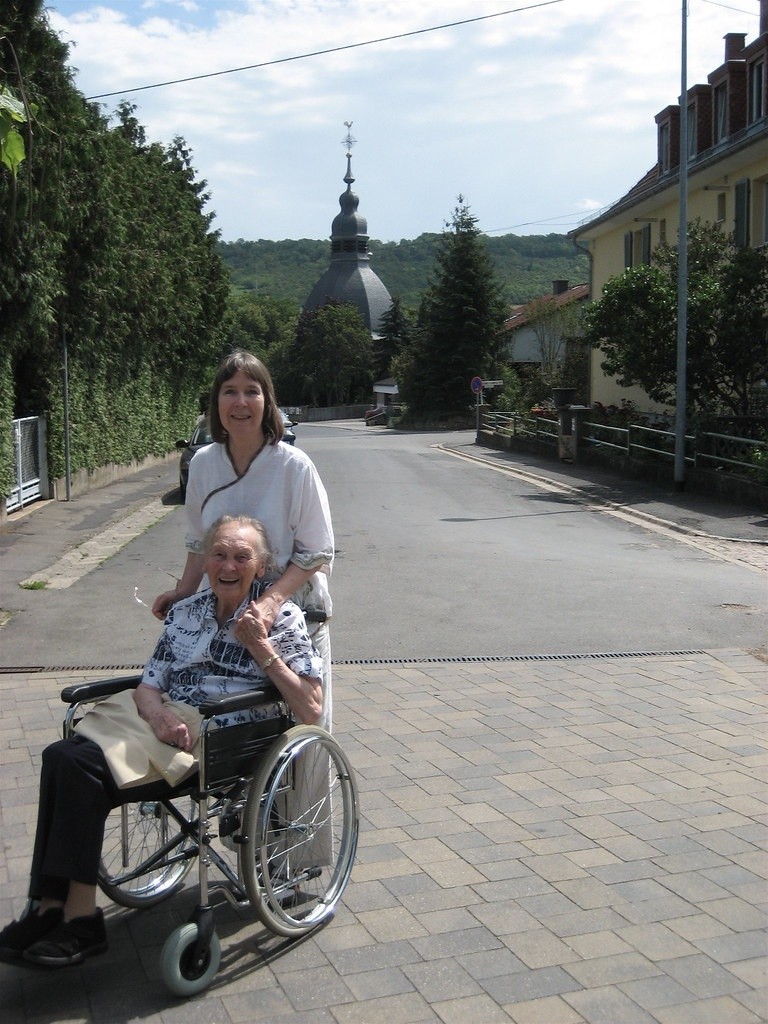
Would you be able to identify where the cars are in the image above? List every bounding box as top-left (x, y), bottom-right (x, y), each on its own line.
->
top-left (175, 409), bottom-right (298, 501)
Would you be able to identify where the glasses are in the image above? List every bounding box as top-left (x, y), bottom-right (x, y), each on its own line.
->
top-left (133, 561), bottom-right (184, 616)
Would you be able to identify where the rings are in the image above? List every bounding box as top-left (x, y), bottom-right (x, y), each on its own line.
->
top-left (170, 742), bottom-right (175, 746)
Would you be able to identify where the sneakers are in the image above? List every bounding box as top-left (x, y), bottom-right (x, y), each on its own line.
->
top-left (1, 907), bottom-right (64, 962)
top-left (24, 906), bottom-right (110, 966)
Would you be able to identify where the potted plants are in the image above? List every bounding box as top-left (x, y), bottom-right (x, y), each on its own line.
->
top-left (549, 365), bottom-right (577, 405)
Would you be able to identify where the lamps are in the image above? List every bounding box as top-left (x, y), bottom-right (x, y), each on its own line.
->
top-left (703, 185), bottom-right (731, 191)
top-left (634, 217), bottom-right (658, 222)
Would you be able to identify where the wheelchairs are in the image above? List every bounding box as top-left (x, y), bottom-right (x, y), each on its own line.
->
top-left (62, 611), bottom-right (361, 999)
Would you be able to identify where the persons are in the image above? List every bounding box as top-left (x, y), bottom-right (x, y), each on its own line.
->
top-left (152, 349), bottom-right (335, 908)
top-left (0, 512), bottom-right (323, 968)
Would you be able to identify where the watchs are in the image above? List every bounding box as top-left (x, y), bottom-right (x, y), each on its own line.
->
top-left (261, 654), bottom-right (280, 671)
